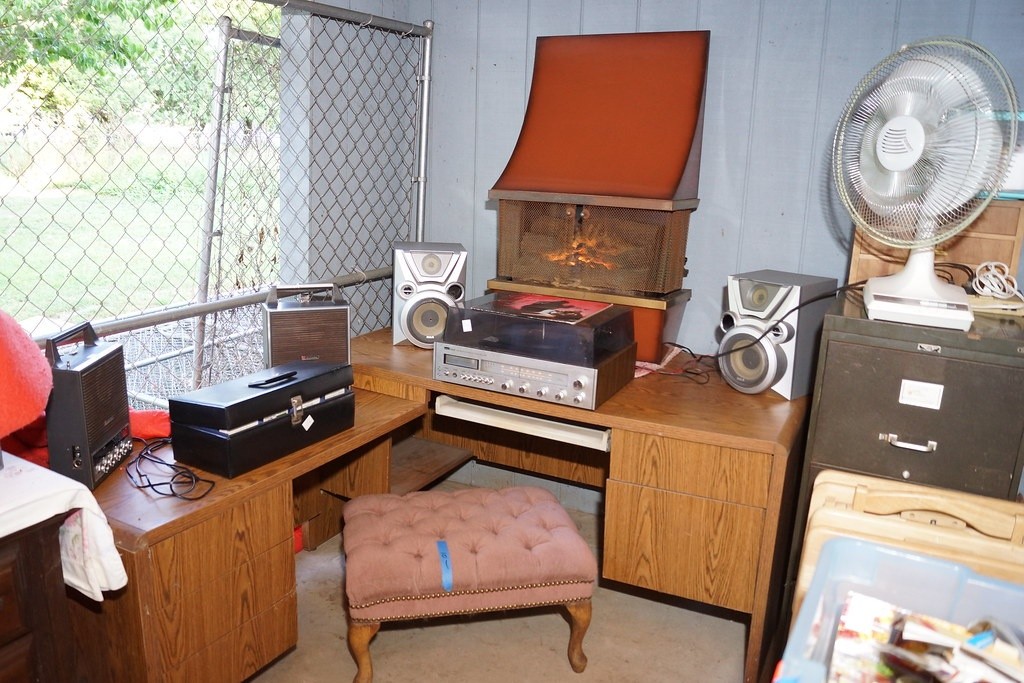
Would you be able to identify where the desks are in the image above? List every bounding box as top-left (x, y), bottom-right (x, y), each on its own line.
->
top-left (0, 450), bottom-right (129, 683)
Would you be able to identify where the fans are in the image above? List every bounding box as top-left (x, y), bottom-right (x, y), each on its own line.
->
top-left (829, 37), bottom-right (1022, 331)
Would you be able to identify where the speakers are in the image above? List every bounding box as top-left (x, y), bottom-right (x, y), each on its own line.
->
top-left (262, 281), bottom-right (351, 366)
top-left (721, 269), bottom-right (838, 401)
top-left (392, 241), bottom-right (469, 349)
top-left (41, 320), bottom-right (133, 491)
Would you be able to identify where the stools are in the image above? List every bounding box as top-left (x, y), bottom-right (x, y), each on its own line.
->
top-left (344, 485), bottom-right (598, 683)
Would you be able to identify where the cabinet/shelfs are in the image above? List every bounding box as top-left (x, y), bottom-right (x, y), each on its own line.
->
top-left (68, 327), bottom-right (810, 683)
top-left (777, 298), bottom-right (1023, 638)
